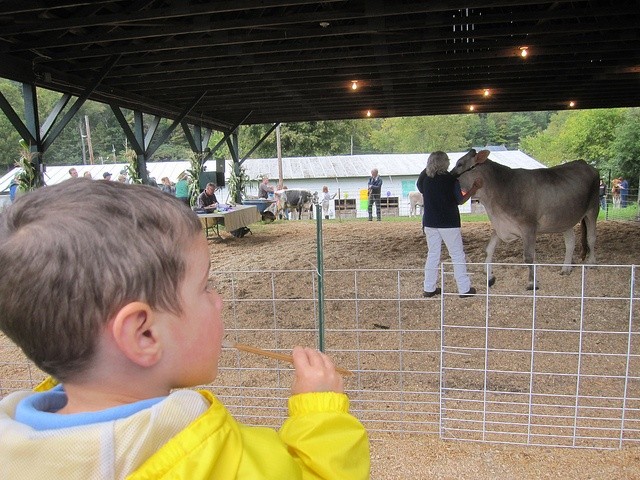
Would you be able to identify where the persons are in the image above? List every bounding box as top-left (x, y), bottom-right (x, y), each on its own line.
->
top-left (176, 176), bottom-right (190, 202)
top-left (198, 183), bottom-right (250, 237)
top-left (0, 178), bottom-right (371, 480)
top-left (416, 151), bottom-right (482, 298)
top-left (10, 173), bottom-right (26, 200)
top-left (599, 176), bottom-right (607, 210)
top-left (619, 176), bottom-right (628, 208)
top-left (368, 169), bottom-right (383, 221)
top-left (161, 177), bottom-right (172, 192)
top-left (319, 186), bottom-right (337, 219)
top-left (258, 177), bottom-right (275, 199)
top-left (276, 179), bottom-right (289, 220)
top-left (69, 168), bottom-right (78, 177)
top-left (118, 175), bottom-right (125, 182)
top-left (611, 179), bottom-right (624, 210)
top-left (103, 172), bottom-right (112, 180)
top-left (84, 171), bottom-right (91, 177)
top-left (120, 171), bottom-right (129, 183)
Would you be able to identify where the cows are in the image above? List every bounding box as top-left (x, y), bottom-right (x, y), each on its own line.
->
top-left (407, 191), bottom-right (424, 217)
top-left (274, 189), bottom-right (319, 220)
top-left (611, 178), bottom-right (622, 210)
top-left (449, 148), bottom-right (601, 291)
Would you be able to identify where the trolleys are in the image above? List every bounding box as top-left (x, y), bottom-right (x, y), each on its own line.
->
top-left (242, 198), bottom-right (277, 222)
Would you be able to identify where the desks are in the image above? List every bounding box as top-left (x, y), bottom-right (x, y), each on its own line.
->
top-left (197, 205), bottom-right (257, 240)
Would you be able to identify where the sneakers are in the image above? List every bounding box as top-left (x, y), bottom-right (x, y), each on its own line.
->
top-left (236, 227), bottom-right (249, 238)
top-left (423, 288), bottom-right (441, 297)
top-left (459, 288), bottom-right (476, 298)
